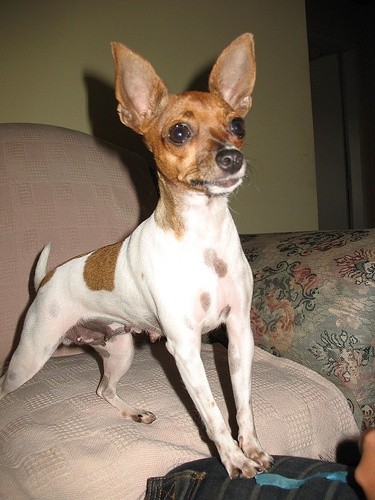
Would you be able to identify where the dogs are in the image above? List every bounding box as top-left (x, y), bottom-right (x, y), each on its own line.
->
top-left (0, 32), bottom-right (276, 479)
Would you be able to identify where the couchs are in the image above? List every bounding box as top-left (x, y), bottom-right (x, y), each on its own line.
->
top-left (0, 123), bottom-right (375, 500)
